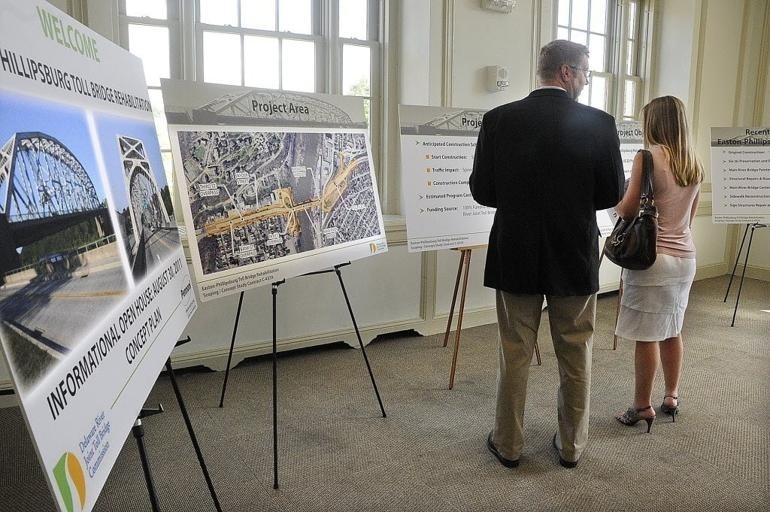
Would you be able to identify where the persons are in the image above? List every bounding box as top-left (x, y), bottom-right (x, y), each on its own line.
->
top-left (464, 37), bottom-right (626, 472)
top-left (610, 93), bottom-right (707, 436)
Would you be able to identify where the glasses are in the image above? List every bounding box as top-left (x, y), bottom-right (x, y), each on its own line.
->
top-left (569, 65), bottom-right (592, 78)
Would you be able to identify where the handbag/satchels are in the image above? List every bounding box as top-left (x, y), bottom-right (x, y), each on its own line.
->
top-left (603, 148), bottom-right (659, 271)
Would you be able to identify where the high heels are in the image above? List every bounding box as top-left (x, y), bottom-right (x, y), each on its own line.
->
top-left (661, 395), bottom-right (679, 422)
top-left (614, 405), bottom-right (656, 434)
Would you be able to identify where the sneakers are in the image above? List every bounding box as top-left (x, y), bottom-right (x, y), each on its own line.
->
top-left (553, 430), bottom-right (577, 467)
top-left (487, 430), bottom-right (520, 467)
